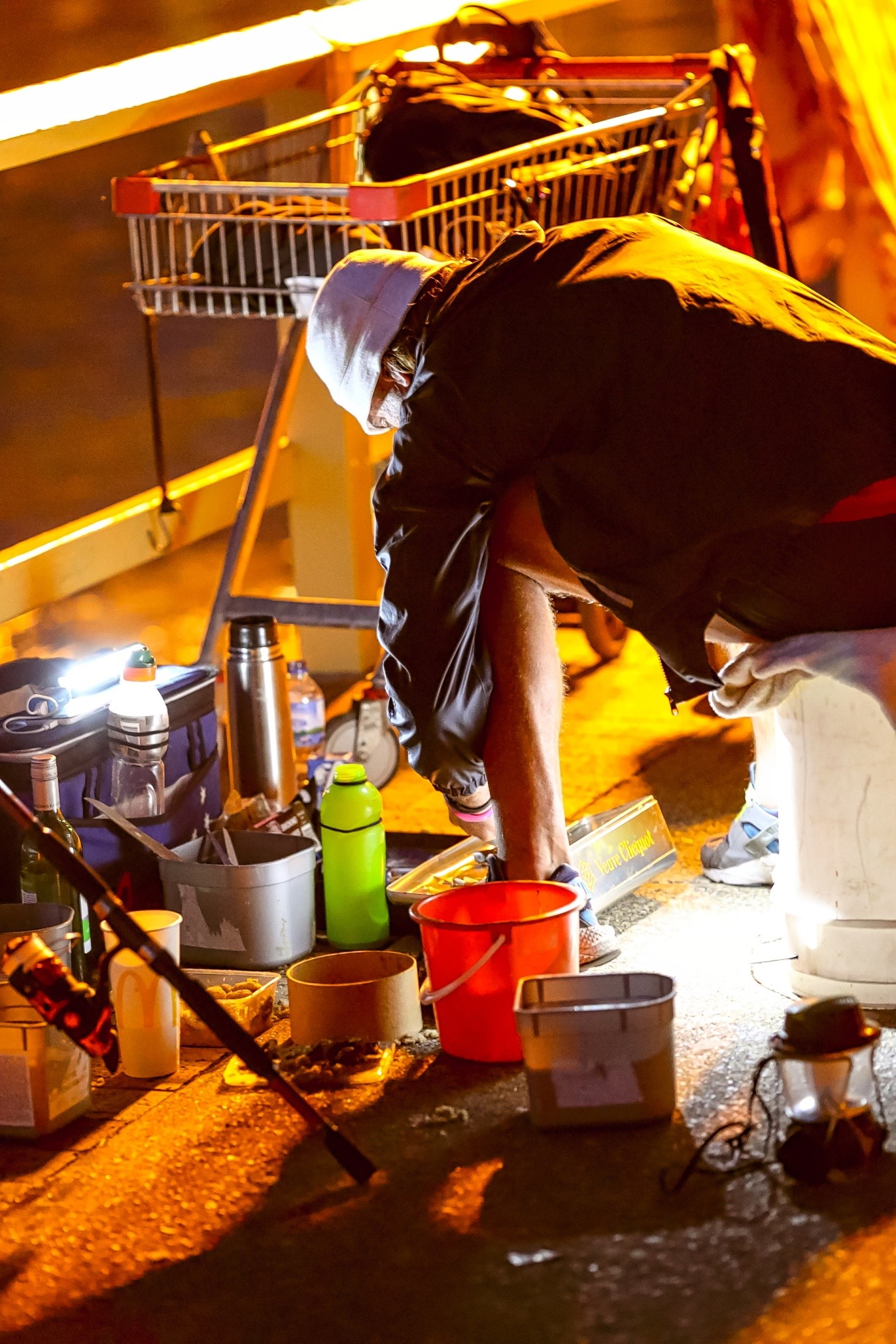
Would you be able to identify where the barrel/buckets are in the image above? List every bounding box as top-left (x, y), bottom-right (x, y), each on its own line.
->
top-left (0, 981), bottom-right (91, 1138)
top-left (514, 970), bottom-right (678, 1129)
top-left (0, 902), bottom-right (81, 980)
top-left (409, 880), bottom-right (587, 1063)
top-left (158, 828), bottom-right (319, 970)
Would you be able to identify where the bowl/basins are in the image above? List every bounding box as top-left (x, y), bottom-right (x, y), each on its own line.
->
top-left (286, 951), bottom-right (423, 1045)
top-left (180, 968), bottom-right (282, 1047)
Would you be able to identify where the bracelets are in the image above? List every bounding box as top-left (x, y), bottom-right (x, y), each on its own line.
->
top-left (443, 794), bottom-right (493, 822)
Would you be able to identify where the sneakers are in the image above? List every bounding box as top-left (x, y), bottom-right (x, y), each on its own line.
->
top-left (416, 852), bottom-right (624, 997)
top-left (700, 760), bottom-right (781, 887)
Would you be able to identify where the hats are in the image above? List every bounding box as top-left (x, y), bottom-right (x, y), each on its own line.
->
top-left (303, 247), bottom-right (461, 441)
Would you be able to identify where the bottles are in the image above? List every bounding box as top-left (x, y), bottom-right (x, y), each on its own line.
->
top-left (320, 763), bottom-right (391, 951)
top-left (286, 660), bottom-right (327, 788)
top-left (20, 754), bottom-right (93, 983)
top-left (107, 647), bottom-right (170, 819)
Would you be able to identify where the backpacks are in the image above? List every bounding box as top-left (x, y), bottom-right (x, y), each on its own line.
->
top-left (348, 49), bottom-right (609, 216)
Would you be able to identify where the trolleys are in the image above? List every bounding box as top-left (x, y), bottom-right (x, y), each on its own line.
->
top-left (96, 42), bottom-right (759, 787)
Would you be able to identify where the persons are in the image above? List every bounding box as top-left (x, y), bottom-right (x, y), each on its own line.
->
top-left (305, 212), bottom-right (896, 970)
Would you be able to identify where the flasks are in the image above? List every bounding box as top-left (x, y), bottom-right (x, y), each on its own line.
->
top-left (226, 615), bottom-right (297, 806)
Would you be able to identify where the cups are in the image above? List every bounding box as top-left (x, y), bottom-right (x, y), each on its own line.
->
top-left (100, 910), bottom-right (184, 1079)
